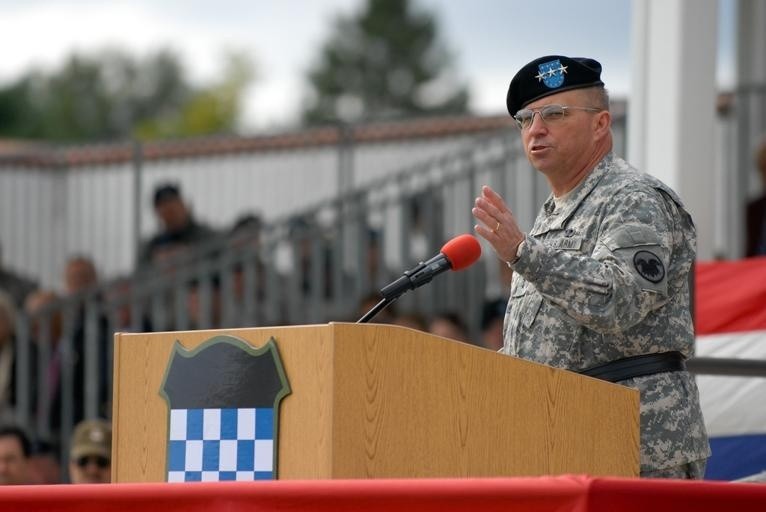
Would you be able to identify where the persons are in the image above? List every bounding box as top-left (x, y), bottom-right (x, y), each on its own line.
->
top-left (0, 255), bottom-right (156, 483)
top-left (136, 182), bottom-right (507, 351)
top-left (469, 53), bottom-right (713, 480)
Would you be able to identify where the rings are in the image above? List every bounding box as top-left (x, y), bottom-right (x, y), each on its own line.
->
top-left (495, 221), bottom-right (502, 232)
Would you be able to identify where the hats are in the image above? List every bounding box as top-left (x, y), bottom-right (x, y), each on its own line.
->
top-left (68, 420), bottom-right (111, 461)
top-left (507, 55), bottom-right (605, 120)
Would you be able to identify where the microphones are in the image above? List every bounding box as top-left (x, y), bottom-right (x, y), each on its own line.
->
top-left (379, 233), bottom-right (481, 298)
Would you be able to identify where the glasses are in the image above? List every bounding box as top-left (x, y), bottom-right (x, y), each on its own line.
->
top-left (512, 104), bottom-right (602, 130)
top-left (77, 456), bottom-right (111, 468)
